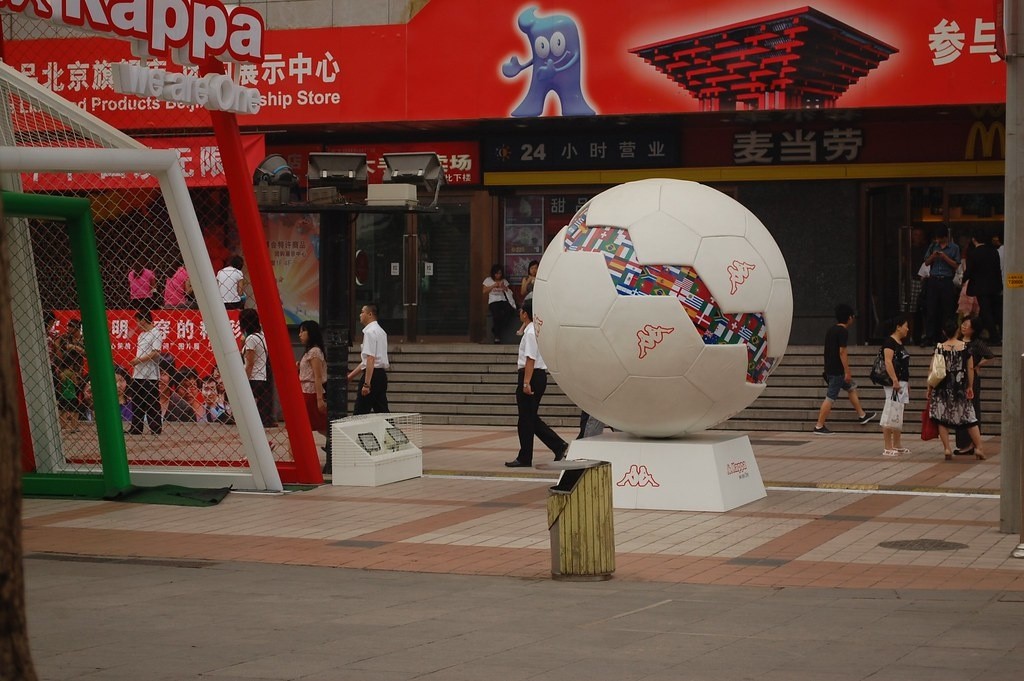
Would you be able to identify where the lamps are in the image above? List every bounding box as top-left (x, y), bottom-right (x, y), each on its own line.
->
top-left (382, 152), bottom-right (445, 208)
top-left (251, 153), bottom-right (300, 205)
top-left (306, 152), bottom-right (368, 205)
top-left (357, 432), bottom-right (381, 453)
top-left (383, 427), bottom-right (409, 449)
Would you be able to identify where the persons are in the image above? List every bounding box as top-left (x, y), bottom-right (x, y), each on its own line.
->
top-left (41, 308), bottom-right (89, 434)
top-left (899, 226), bottom-right (1003, 348)
top-left (123, 310), bottom-right (163, 435)
top-left (575, 410), bottom-right (622, 440)
top-left (814, 303), bottom-right (877, 435)
top-left (954, 315), bottom-right (995, 456)
top-left (288, 320), bottom-right (328, 462)
top-left (516, 261), bottom-right (538, 336)
top-left (127, 255), bottom-right (246, 311)
top-left (482, 265), bottom-right (514, 344)
top-left (80, 352), bottom-right (237, 424)
top-left (238, 308), bottom-right (275, 453)
top-left (505, 304), bottom-right (569, 467)
top-left (321, 304), bottom-right (390, 453)
top-left (880, 316), bottom-right (912, 457)
top-left (925, 317), bottom-right (987, 461)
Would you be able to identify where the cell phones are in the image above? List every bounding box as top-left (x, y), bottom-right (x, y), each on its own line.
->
top-left (937, 252), bottom-right (942, 254)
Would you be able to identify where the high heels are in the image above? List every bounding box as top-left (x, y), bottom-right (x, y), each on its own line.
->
top-left (945, 448), bottom-right (952, 460)
top-left (974, 448), bottom-right (987, 460)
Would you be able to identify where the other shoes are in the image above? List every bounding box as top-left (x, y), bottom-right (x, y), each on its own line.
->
top-left (554, 442), bottom-right (569, 461)
top-left (268, 440), bottom-right (275, 451)
top-left (321, 445), bottom-right (325, 452)
top-left (516, 330), bottom-right (524, 337)
top-left (505, 459), bottom-right (532, 467)
top-left (920, 339), bottom-right (935, 348)
top-left (123, 430), bottom-right (140, 435)
top-left (954, 447), bottom-right (974, 455)
top-left (494, 337), bottom-right (501, 344)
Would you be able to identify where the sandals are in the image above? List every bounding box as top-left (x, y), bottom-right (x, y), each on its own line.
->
top-left (892, 447), bottom-right (912, 454)
top-left (882, 449), bottom-right (898, 457)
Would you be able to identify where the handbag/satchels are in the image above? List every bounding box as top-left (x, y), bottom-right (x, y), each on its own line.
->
top-left (927, 342), bottom-right (947, 388)
top-left (504, 288), bottom-right (517, 310)
top-left (921, 394), bottom-right (940, 441)
top-left (869, 340), bottom-right (901, 386)
top-left (879, 389), bottom-right (904, 431)
top-left (823, 372), bottom-right (829, 385)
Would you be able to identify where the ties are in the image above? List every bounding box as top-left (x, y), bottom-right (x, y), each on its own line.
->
top-left (205, 406), bottom-right (213, 423)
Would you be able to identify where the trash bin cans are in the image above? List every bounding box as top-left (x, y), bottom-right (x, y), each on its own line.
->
top-left (546, 459), bottom-right (616, 583)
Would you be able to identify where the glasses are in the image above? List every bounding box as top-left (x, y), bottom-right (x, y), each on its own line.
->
top-left (179, 382), bottom-right (197, 390)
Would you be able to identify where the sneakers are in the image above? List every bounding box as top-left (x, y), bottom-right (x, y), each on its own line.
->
top-left (859, 412), bottom-right (877, 424)
top-left (813, 425), bottom-right (836, 436)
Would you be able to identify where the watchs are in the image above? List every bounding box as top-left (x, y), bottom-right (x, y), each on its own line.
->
top-left (523, 384), bottom-right (530, 387)
top-left (363, 382), bottom-right (370, 388)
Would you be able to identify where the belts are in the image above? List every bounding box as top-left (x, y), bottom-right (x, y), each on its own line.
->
top-left (931, 275), bottom-right (953, 279)
